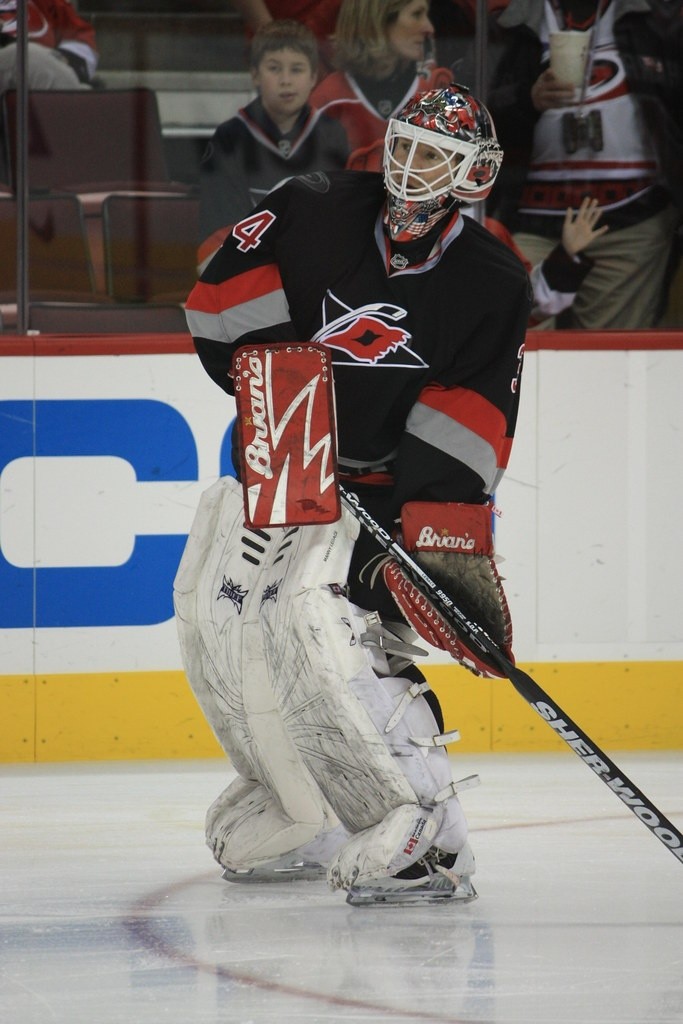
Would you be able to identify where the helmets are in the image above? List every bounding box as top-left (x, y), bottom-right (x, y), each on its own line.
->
top-left (383, 81), bottom-right (504, 213)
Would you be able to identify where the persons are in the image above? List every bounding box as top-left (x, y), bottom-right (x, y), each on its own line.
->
top-left (0, 0), bottom-right (99, 91)
top-left (241, 0), bottom-right (342, 83)
top-left (488, 0), bottom-right (683, 331)
top-left (196, 20), bottom-right (349, 264)
top-left (312, 0), bottom-right (455, 173)
top-left (488, 197), bottom-right (608, 329)
top-left (170, 83), bottom-right (529, 904)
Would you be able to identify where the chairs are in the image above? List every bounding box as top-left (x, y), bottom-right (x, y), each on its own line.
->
top-left (0, 75), bottom-right (208, 338)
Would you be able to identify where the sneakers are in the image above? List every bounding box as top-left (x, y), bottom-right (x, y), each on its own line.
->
top-left (345, 848), bottom-right (479, 911)
